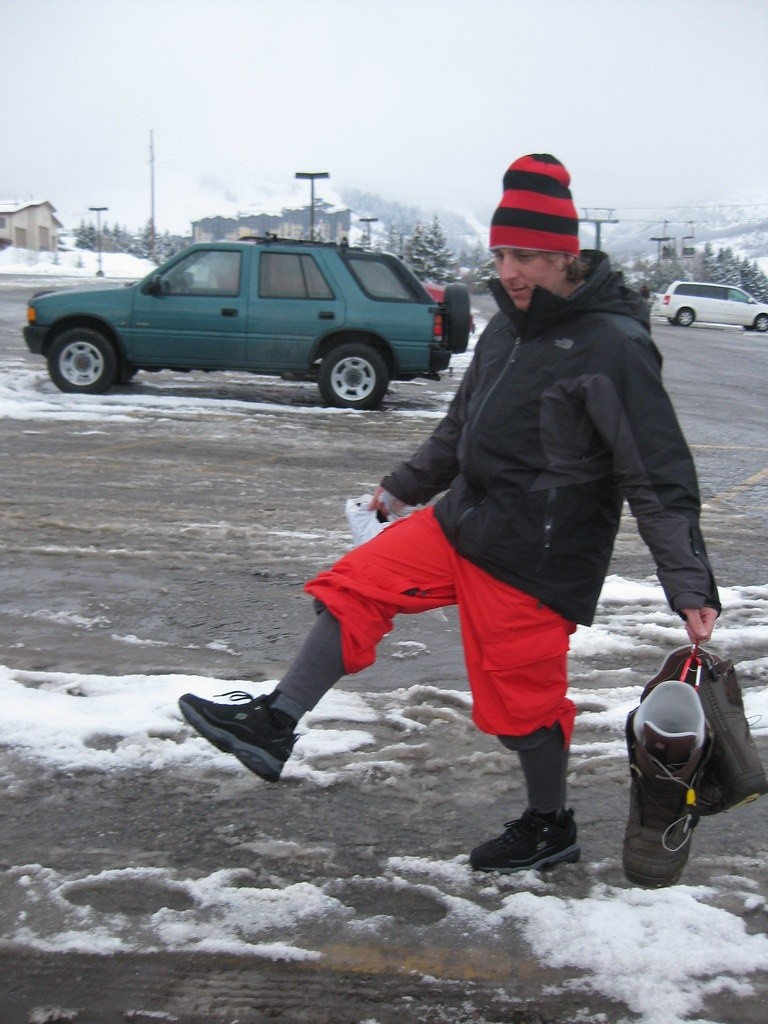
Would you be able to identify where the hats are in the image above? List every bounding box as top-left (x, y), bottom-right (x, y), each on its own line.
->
top-left (489, 154), bottom-right (580, 257)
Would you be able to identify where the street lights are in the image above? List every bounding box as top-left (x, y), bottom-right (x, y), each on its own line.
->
top-left (89, 207), bottom-right (109, 276)
top-left (295, 172), bottom-right (329, 239)
top-left (361, 218), bottom-right (379, 244)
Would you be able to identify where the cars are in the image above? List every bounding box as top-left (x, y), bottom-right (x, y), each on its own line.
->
top-left (658, 279), bottom-right (768, 332)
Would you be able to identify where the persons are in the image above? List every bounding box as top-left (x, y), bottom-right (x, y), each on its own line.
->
top-left (181, 153), bottom-right (721, 874)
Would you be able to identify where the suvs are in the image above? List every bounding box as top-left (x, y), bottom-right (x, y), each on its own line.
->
top-left (23, 228), bottom-right (474, 411)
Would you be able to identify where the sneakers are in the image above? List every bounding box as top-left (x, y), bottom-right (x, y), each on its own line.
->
top-left (179, 691), bottom-right (301, 783)
top-left (622, 681), bottom-right (714, 888)
top-left (639, 645), bottom-right (768, 817)
top-left (470, 807), bottom-right (581, 875)
